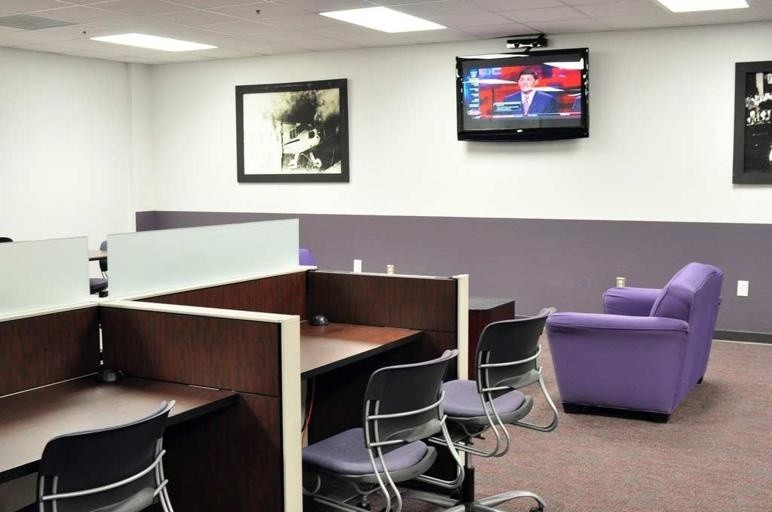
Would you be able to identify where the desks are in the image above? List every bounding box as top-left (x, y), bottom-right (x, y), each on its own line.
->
top-left (108, 264), bottom-right (470, 511)
top-left (470, 296), bottom-right (516, 380)
top-left (1, 298), bottom-right (303, 512)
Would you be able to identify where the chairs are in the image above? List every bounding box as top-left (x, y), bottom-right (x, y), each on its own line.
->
top-left (545, 262), bottom-right (724, 422)
top-left (300, 348), bottom-right (464, 512)
top-left (36, 400), bottom-right (175, 512)
top-left (435, 306), bottom-right (559, 512)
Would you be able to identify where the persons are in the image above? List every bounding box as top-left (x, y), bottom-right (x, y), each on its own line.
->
top-left (500, 68), bottom-right (560, 116)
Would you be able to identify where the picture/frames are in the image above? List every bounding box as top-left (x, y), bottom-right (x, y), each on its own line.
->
top-left (732, 60), bottom-right (771, 186)
top-left (234, 78), bottom-right (351, 186)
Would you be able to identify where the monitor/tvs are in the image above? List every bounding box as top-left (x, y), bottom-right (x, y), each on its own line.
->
top-left (455, 54), bottom-right (592, 142)
